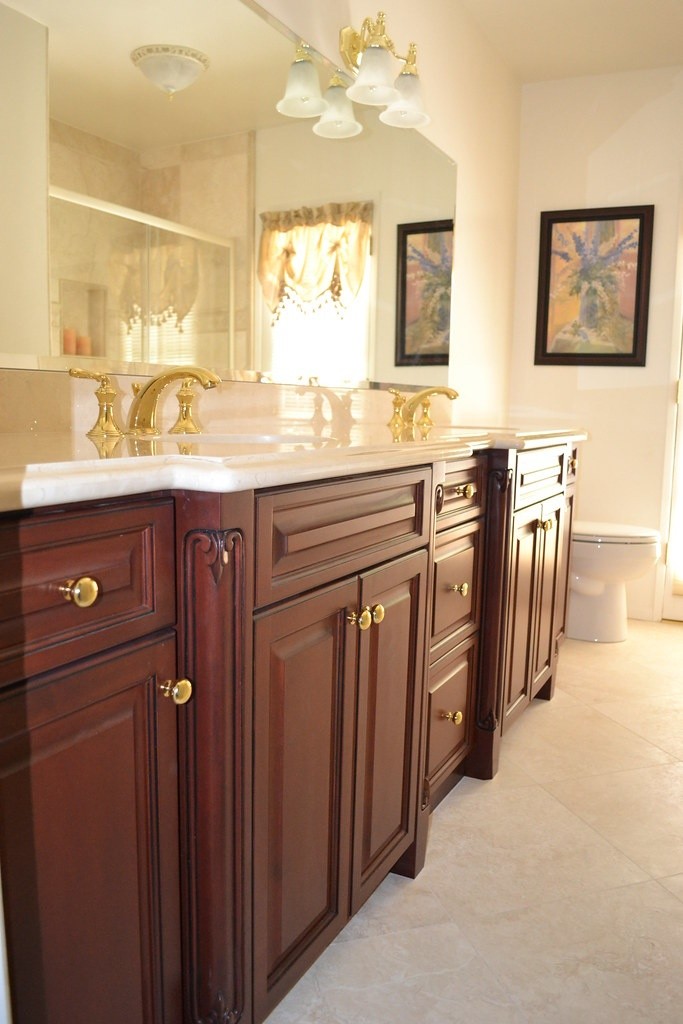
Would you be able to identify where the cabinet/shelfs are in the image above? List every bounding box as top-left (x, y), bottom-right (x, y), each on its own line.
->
top-left (554, 441), bottom-right (580, 653)
top-left (500, 442), bottom-right (568, 735)
top-left (0, 490), bottom-right (190, 1024)
top-left (252, 465), bottom-right (433, 1022)
top-left (429, 453), bottom-right (488, 795)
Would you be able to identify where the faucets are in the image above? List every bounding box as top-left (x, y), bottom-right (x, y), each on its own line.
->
top-left (123, 365), bottom-right (223, 438)
top-left (400, 385), bottom-right (461, 429)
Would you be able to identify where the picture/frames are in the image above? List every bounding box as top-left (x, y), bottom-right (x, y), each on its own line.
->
top-left (532, 204), bottom-right (654, 367)
top-left (395, 219), bottom-right (455, 367)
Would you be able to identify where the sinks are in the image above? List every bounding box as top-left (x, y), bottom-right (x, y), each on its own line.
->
top-left (144, 430), bottom-right (338, 447)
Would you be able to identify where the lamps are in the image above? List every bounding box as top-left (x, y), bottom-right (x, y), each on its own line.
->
top-left (337, 9), bottom-right (432, 128)
top-left (130, 43), bottom-right (214, 104)
top-left (274, 43), bottom-right (362, 139)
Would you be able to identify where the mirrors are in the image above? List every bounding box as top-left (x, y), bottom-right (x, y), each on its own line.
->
top-left (0, 0), bottom-right (456, 395)
